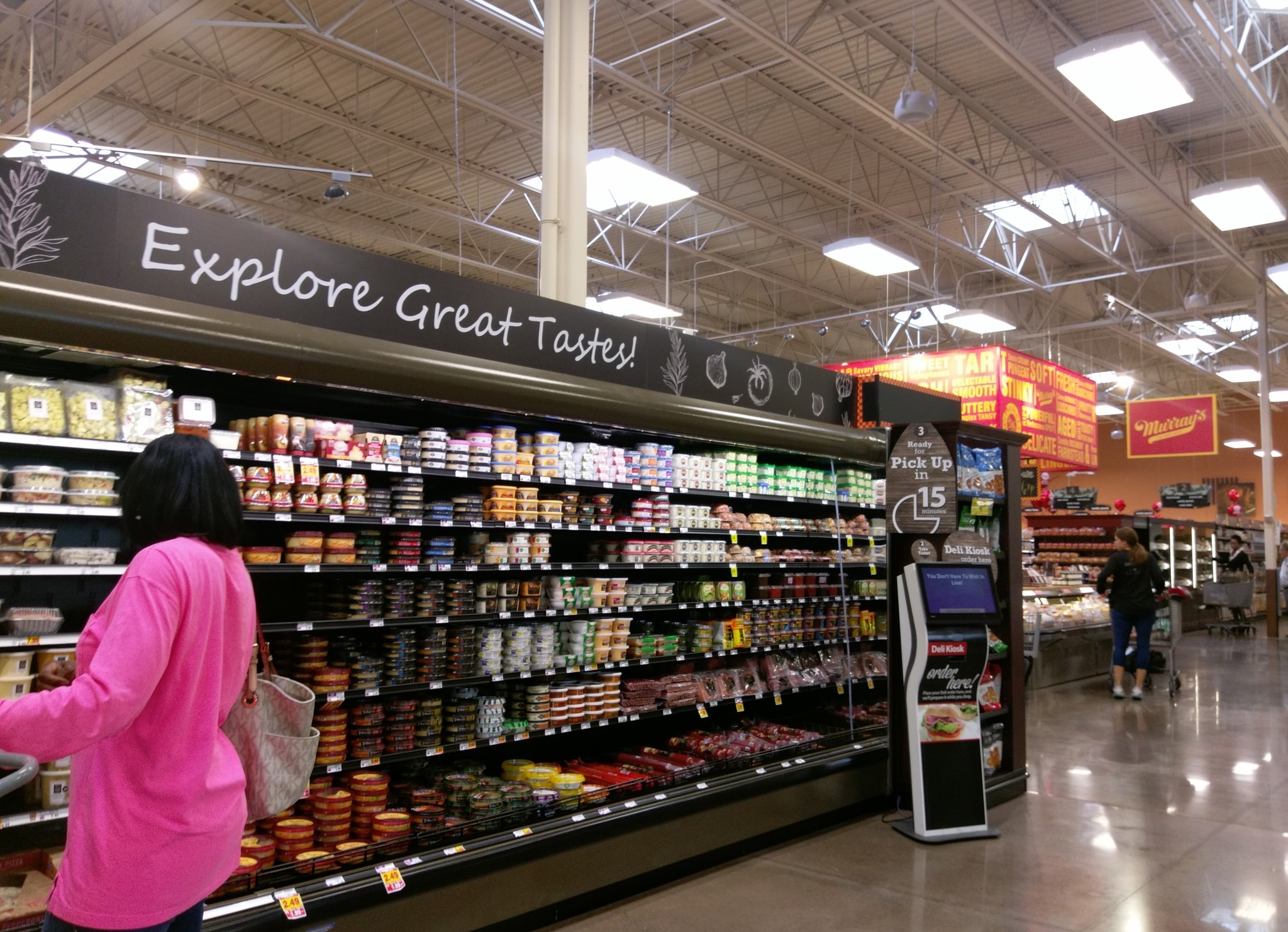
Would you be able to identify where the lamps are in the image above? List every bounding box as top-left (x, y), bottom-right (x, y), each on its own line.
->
top-left (1077, 306), bottom-right (1288, 461)
top-left (1187, 175), bottom-right (1287, 233)
top-left (1050, 28), bottom-right (1197, 125)
top-left (888, 0), bottom-right (937, 126)
top-left (1265, 260), bottom-right (1288, 298)
top-left (2, 116), bottom-right (1163, 358)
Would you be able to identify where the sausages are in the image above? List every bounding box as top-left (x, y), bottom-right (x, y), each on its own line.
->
top-left (620, 674), bottom-right (698, 716)
top-left (832, 700), bottom-right (888, 725)
top-left (555, 720), bottom-right (825, 791)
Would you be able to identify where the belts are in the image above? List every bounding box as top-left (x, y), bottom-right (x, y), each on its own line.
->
top-left (1280, 586), bottom-right (1288, 592)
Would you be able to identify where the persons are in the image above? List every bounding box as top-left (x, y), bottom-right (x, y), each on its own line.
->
top-left (0, 434), bottom-right (258, 932)
top-left (1150, 534), bottom-right (1169, 583)
top-left (1211, 534), bottom-right (1255, 634)
top-left (1097, 527), bottom-right (1166, 702)
top-left (1278, 542), bottom-right (1288, 638)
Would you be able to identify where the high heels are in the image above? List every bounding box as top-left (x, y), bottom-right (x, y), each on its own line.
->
top-left (1230, 620), bottom-right (1251, 634)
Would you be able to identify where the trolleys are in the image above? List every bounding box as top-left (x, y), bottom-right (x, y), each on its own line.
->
top-left (1201, 574), bottom-right (1257, 637)
top-left (1108, 593), bottom-right (1182, 697)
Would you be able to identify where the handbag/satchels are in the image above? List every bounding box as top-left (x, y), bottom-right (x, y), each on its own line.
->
top-left (220, 606), bottom-right (321, 826)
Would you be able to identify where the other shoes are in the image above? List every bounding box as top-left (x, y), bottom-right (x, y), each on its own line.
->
top-left (1112, 686), bottom-right (1126, 699)
top-left (1132, 686), bottom-right (1143, 699)
